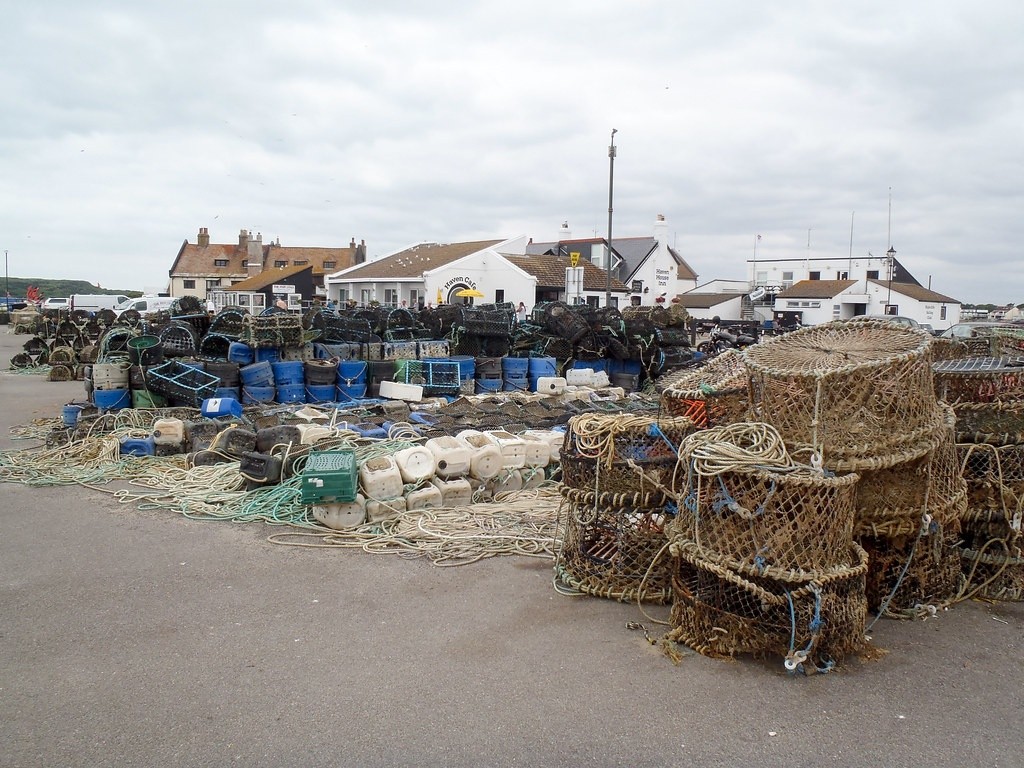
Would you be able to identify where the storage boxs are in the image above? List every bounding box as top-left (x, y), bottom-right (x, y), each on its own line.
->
top-left (301, 450), bottom-right (357, 504)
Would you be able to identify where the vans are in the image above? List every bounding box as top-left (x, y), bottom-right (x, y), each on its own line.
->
top-left (72, 295), bottom-right (131, 312)
top-left (112, 294), bottom-right (182, 316)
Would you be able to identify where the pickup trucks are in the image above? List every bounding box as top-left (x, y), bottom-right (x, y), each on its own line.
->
top-left (44, 297), bottom-right (71, 310)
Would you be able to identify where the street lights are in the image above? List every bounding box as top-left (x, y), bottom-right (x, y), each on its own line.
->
top-left (4, 249), bottom-right (9, 312)
top-left (606, 129), bottom-right (618, 307)
top-left (887, 246), bottom-right (896, 314)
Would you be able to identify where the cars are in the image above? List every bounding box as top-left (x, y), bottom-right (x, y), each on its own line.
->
top-left (850, 314), bottom-right (937, 336)
top-left (24, 299), bottom-right (41, 307)
top-left (938, 321), bottom-right (1024, 337)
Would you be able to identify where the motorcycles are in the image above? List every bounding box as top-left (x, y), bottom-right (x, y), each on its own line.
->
top-left (697, 314), bottom-right (757, 352)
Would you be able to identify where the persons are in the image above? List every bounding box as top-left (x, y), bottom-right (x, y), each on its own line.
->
top-left (400, 299), bottom-right (433, 310)
top-left (517, 302), bottom-right (526, 322)
top-left (206, 300), bottom-right (214, 314)
top-left (275, 297), bottom-right (358, 311)
top-left (771, 314), bottom-right (802, 336)
